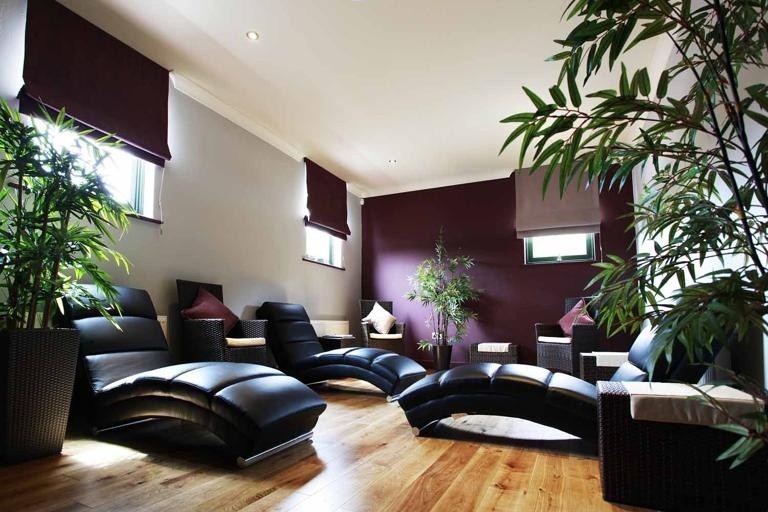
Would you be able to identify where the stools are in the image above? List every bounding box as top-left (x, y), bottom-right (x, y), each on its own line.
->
top-left (319, 335), bottom-right (356, 351)
top-left (595, 381), bottom-right (768, 511)
top-left (469, 343), bottom-right (521, 364)
top-left (579, 351), bottom-right (629, 386)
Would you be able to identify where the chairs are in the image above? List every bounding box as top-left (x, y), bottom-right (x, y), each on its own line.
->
top-left (168, 279), bottom-right (268, 364)
top-left (534, 296), bottom-right (601, 374)
top-left (399, 289), bottom-right (735, 448)
top-left (256, 302), bottom-right (427, 403)
top-left (51, 282), bottom-right (326, 469)
top-left (359, 300), bottom-right (406, 356)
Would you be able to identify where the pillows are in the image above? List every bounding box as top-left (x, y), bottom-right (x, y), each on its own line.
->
top-left (180, 287), bottom-right (240, 337)
top-left (558, 299), bottom-right (596, 337)
top-left (361, 301), bottom-right (397, 335)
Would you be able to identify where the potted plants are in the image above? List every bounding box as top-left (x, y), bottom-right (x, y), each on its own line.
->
top-left (0, 96), bottom-right (139, 461)
top-left (404, 222), bottom-right (488, 369)
